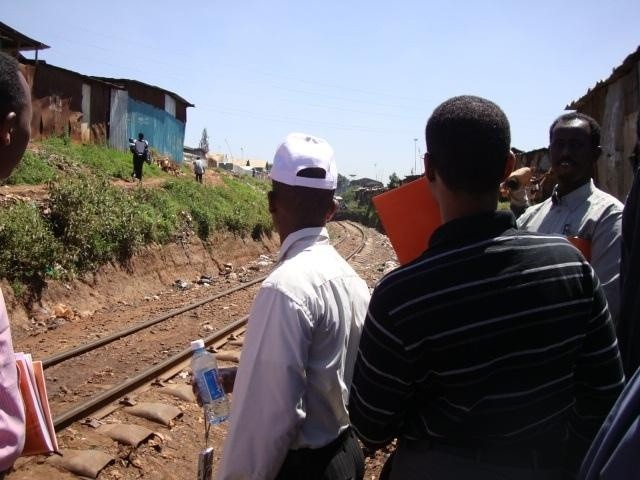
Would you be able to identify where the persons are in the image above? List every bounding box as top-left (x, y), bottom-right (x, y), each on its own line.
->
top-left (193, 156), bottom-right (204, 183)
top-left (129, 138), bottom-right (143, 153)
top-left (0, 51), bottom-right (33, 480)
top-left (133, 133), bottom-right (150, 179)
top-left (190, 135), bottom-right (372, 480)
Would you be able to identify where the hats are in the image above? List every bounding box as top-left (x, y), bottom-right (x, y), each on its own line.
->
top-left (267, 134), bottom-right (338, 191)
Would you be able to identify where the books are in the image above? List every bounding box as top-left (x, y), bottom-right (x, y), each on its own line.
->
top-left (15, 352), bottom-right (58, 456)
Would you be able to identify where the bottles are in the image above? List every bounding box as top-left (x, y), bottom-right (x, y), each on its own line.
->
top-left (189, 338), bottom-right (231, 427)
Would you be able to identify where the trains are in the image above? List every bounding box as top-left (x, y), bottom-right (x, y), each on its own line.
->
top-left (335, 195), bottom-right (350, 222)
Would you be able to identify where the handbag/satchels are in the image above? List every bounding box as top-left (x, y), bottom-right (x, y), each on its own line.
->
top-left (144, 148), bottom-right (151, 163)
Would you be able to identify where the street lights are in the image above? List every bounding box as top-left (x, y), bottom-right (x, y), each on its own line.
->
top-left (413, 137), bottom-right (419, 176)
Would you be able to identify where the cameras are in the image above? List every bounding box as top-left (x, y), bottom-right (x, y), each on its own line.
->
top-left (197, 447), bottom-right (214, 480)
